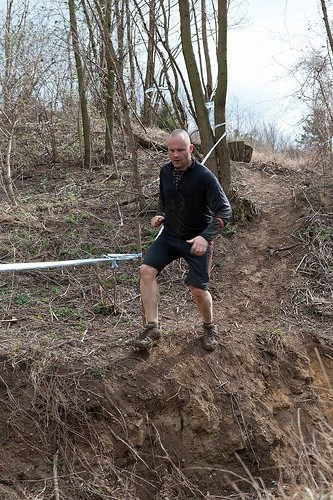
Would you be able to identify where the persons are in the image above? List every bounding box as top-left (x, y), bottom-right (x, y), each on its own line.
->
top-left (134, 127), bottom-right (234, 352)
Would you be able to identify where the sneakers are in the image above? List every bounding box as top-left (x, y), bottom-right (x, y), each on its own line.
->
top-left (135, 323), bottom-right (164, 347)
top-left (201, 322), bottom-right (220, 351)
